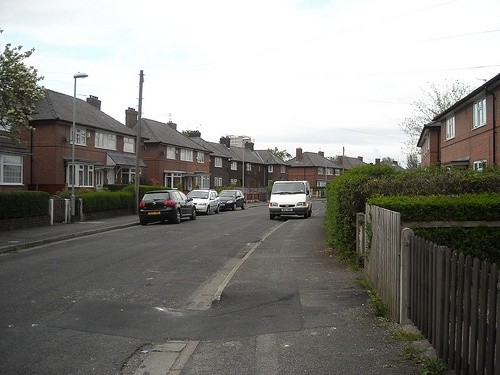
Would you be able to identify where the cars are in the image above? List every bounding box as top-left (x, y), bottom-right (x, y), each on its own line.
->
top-left (138, 190), bottom-right (197, 226)
top-left (186, 189), bottom-right (220, 215)
top-left (218, 190), bottom-right (246, 211)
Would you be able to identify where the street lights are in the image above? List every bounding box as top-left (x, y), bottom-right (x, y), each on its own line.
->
top-left (71, 71), bottom-right (88, 225)
top-left (243, 138), bottom-right (249, 192)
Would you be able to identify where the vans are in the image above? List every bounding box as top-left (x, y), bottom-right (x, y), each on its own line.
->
top-left (269, 180), bottom-right (313, 219)
